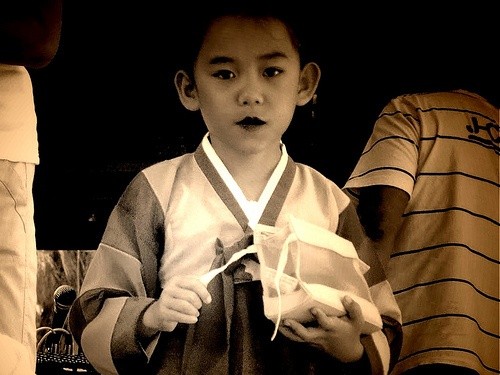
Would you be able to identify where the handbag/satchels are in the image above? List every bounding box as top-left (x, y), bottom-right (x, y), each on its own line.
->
top-left (251, 220), bottom-right (385, 333)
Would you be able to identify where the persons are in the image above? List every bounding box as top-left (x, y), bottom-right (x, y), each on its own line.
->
top-left (69, 1), bottom-right (403, 374)
top-left (340, 19), bottom-right (499, 374)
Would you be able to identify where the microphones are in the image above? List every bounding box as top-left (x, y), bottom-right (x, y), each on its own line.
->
top-left (47, 285), bottom-right (77, 344)
